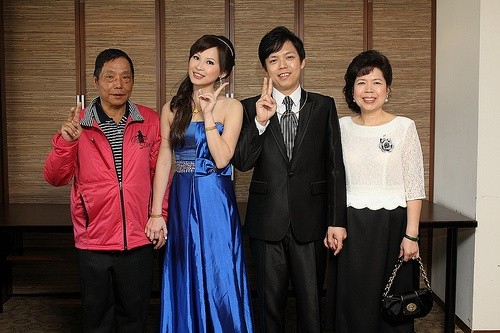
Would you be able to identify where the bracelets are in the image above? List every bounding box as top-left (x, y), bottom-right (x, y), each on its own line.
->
top-left (150, 214), bottom-right (163, 218)
top-left (403, 233), bottom-right (420, 242)
top-left (204, 125), bottom-right (217, 132)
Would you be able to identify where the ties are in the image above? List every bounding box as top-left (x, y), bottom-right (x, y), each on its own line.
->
top-left (280, 96), bottom-right (298, 162)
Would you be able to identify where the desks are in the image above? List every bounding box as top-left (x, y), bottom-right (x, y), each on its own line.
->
top-left (235, 198), bottom-right (478, 333)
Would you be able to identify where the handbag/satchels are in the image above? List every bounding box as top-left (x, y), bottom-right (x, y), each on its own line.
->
top-left (381, 255), bottom-right (433, 320)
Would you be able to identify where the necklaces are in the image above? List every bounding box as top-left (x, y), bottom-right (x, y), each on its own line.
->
top-left (267, 91), bottom-right (308, 116)
top-left (192, 106), bottom-right (203, 116)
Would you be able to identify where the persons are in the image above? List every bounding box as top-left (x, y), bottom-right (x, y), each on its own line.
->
top-left (43, 49), bottom-right (172, 333)
top-left (324, 51), bottom-right (425, 333)
top-left (145, 34), bottom-right (252, 333)
top-left (231, 26), bottom-right (347, 333)
